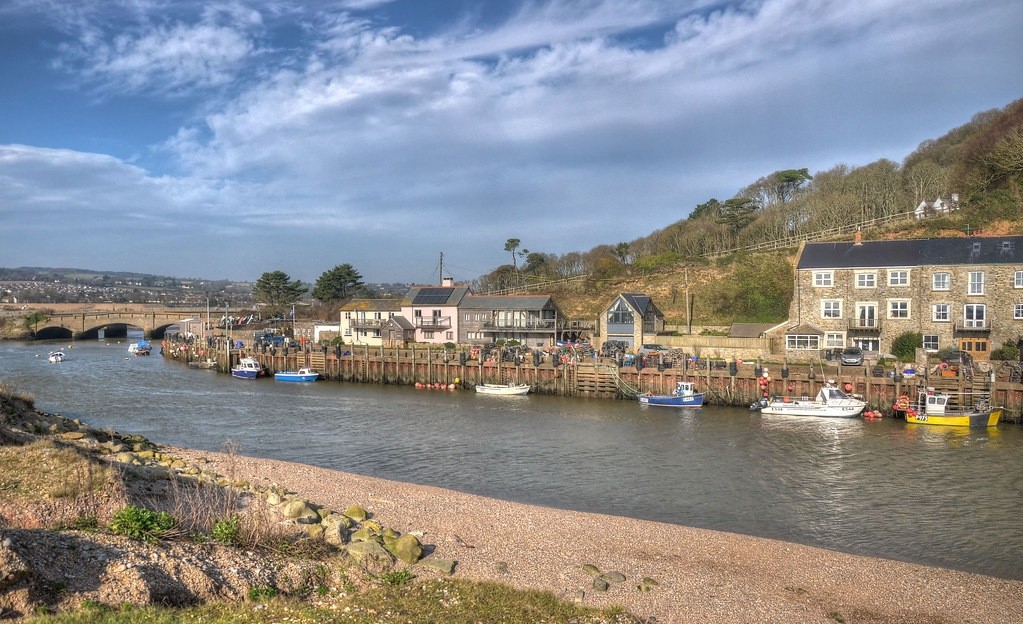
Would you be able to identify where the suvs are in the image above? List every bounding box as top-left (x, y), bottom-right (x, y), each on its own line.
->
top-left (637, 343), bottom-right (670, 359)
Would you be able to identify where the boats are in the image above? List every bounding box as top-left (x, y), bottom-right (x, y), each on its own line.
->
top-left (475, 382), bottom-right (532, 396)
top-left (749, 356), bottom-right (869, 420)
top-left (894, 387), bottom-right (1002, 426)
top-left (129, 340), bottom-right (153, 357)
top-left (634, 381), bottom-right (707, 409)
top-left (49, 352), bottom-right (66, 362)
top-left (231, 357), bottom-right (265, 381)
top-left (274, 368), bottom-right (321, 382)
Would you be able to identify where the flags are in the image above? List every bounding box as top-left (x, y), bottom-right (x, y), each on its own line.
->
top-left (288, 310), bottom-right (293, 317)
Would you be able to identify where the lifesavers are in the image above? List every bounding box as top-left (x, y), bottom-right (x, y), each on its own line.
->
top-left (899, 395), bottom-right (909, 401)
top-left (897, 399), bottom-right (909, 410)
top-left (940, 362), bottom-right (949, 371)
top-left (561, 354), bottom-right (569, 364)
top-left (198, 351), bottom-right (204, 357)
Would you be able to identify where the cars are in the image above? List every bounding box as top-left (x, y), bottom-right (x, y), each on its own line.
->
top-left (942, 350), bottom-right (975, 367)
top-left (839, 347), bottom-right (865, 366)
